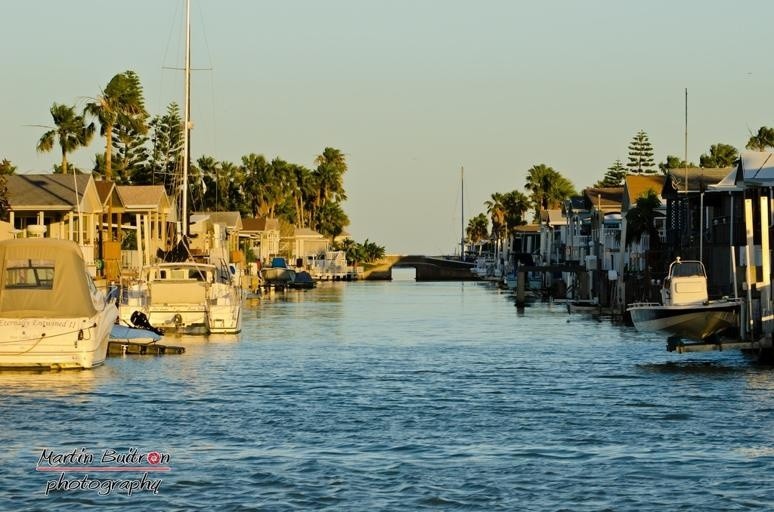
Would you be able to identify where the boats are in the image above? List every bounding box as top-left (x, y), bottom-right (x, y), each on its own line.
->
top-left (625, 193), bottom-right (773, 355)
top-left (0, 240), bottom-right (118, 368)
top-left (261, 258), bottom-right (314, 288)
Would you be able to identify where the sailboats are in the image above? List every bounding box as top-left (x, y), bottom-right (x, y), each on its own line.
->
top-left (118, 1), bottom-right (242, 335)
